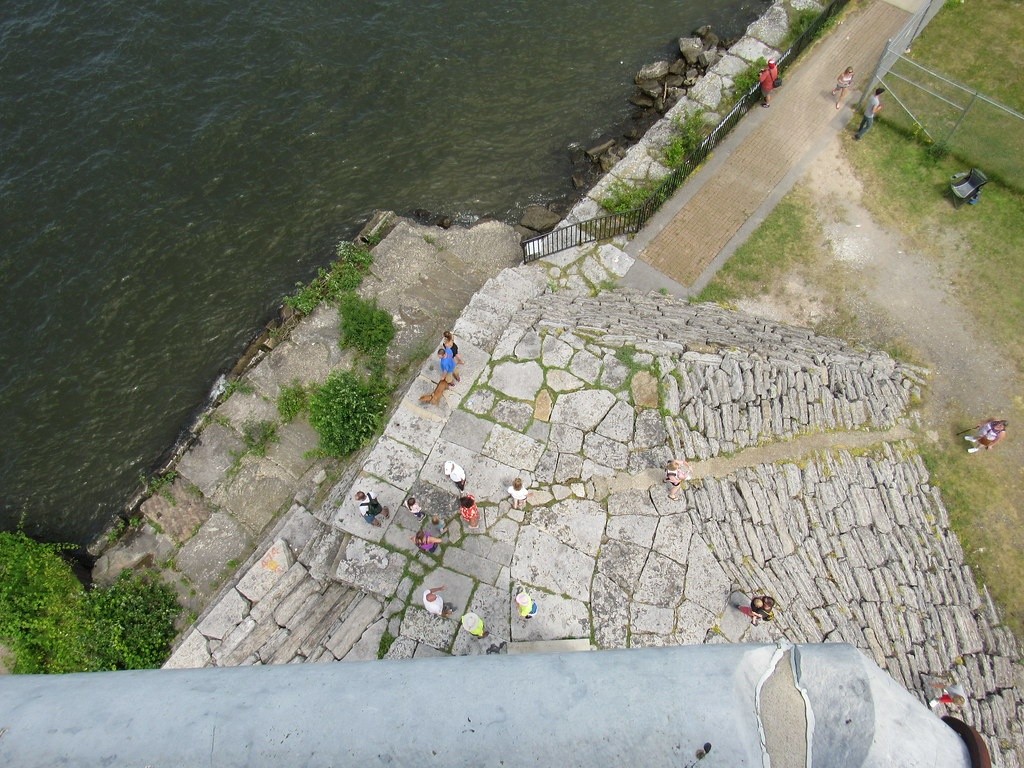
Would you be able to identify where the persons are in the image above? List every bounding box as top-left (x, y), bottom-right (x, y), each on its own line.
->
top-left (516, 592), bottom-right (538, 620)
top-left (444, 461), bottom-right (467, 494)
top-left (964, 417), bottom-right (1009, 454)
top-left (750, 596), bottom-right (780, 627)
top-left (927, 678), bottom-right (966, 706)
top-left (759, 59), bottom-right (778, 108)
top-left (354, 491), bottom-right (390, 527)
top-left (460, 493), bottom-right (482, 529)
top-left (462, 612), bottom-right (489, 639)
top-left (405, 498), bottom-right (444, 555)
top-left (854, 88), bottom-right (886, 142)
top-left (437, 331), bottom-right (465, 387)
top-left (832, 66), bottom-right (855, 109)
top-left (662, 459), bottom-right (690, 502)
top-left (508, 478), bottom-right (528, 509)
top-left (423, 585), bottom-right (457, 616)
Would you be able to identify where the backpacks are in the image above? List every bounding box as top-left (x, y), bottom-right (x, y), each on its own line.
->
top-left (762, 610), bottom-right (775, 622)
top-left (360, 493), bottom-right (382, 516)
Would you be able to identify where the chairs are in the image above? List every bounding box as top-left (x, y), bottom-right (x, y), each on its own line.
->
top-left (944, 168), bottom-right (988, 210)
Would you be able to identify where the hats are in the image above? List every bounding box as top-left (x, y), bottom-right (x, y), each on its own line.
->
top-left (876, 88), bottom-right (885, 95)
top-left (768, 59), bottom-right (776, 69)
top-left (444, 462), bottom-right (453, 475)
top-left (463, 613), bottom-right (479, 631)
top-left (516, 593), bottom-right (530, 605)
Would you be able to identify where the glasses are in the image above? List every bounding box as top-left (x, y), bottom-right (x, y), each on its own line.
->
top-left (848, 71), bottom-right (853, 73)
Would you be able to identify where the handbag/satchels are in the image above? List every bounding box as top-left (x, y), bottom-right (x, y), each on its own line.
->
top-left (978, 436), bottom-right (993, 446)
top-left (773, 78), bottom-right (782, 87)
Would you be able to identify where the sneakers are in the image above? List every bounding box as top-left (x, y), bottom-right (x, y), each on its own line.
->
top-left (964, 435), bottom-right (976, 442)
top-left (967, 448), bottom-right (979, 453)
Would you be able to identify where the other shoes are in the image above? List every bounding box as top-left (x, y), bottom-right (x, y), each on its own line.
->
top-left (668, 495), bottom-right (679, 501)
top-left (382, 506), bottom-right (389, 519)
top-left (837, 103), bottom-right (839, 109)
top-left (663, 479), bottom-right (667, 483)
top-left (456, 377), bottom-right (460, 382)
top-left (833, 89), bottom-right (835, 95)
top-left (371, 518), bottom-right (381, 527)
top-left (447, 383), bottom-right (455, 388)
top-left (855, 134), bottom-right (861, 140)
top-left (468, 524), bottom-right (479, 528)
top-left (761, 103), bottom-right (770, 108)
top-left (419, 514), bottom-right (426, 522)
top-left (764, 98), bottom-right (766, 102)
top-left (413, 514), bottom-right (418, 517)
top-left (751, 620), bottom-right (758, 626)
